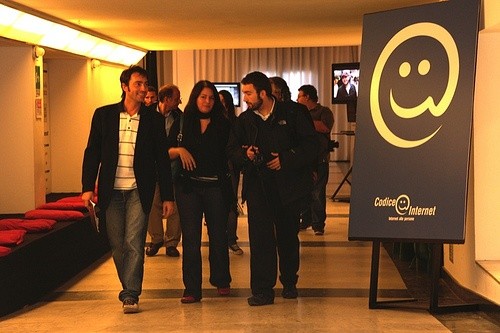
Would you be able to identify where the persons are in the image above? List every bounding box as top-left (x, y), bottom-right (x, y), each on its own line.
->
top-left (218, 90), bottom-right (243, 254)
top-left (296, 85), bottom-right (334, 234)
top-left (267, 77), bottom-right (316, 145)
top-left (237, 70), bottom-right (312, 306)
top-left (334, 73), bottom-right (359, 98)
top-left (144, 85), bottom-right (157, 105)
top-left (81, 64), bottom-right (176, 314)
top-left (167, 80), bottom-right (240, 303)
top-left (146, 84), bottom-right (181, 257)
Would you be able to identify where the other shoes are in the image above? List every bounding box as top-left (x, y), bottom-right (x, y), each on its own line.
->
top-left (123, 299), bottom-right (139, 313)
top-left (314, 228), bottom-right (324, 235)
top-left (282, 284), bottom-right (298, 299)
top-left (247, 294), bottom-right (274, 306)
top-left (229, 243), bottom-right (243, 255)
top-left (166, 246), bottom-right (180, 257)
top-left (146, 242), bottom-right (163, 256)
top-left (217, 286), bottom-right (231, 296)
top-left (181, 295), bottom-right (201, 303)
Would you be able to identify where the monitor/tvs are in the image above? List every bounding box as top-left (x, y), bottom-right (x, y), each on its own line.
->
top-left (212, 83), bottom-right (241, 107)
top-left (332, 62), bottom-right (360, 104)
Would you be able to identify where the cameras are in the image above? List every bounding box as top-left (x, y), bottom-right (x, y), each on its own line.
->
top-left (329, 140), bottom-right (340, 153)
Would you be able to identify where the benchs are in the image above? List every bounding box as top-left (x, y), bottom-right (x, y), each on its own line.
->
top-left (0, 187), bottom-right (110, 317)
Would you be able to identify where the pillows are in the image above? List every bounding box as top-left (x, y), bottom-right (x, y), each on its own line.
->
top-left (0, 187), bottom-right (98, 255)
